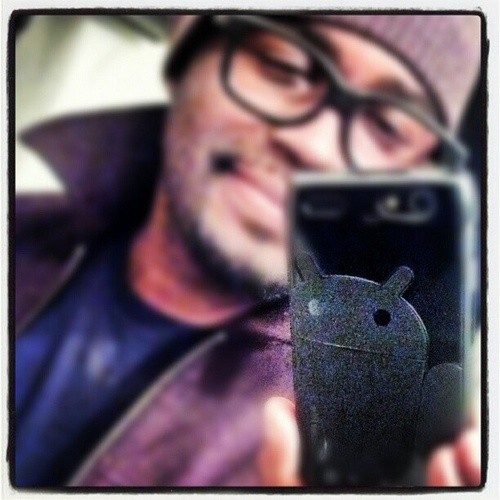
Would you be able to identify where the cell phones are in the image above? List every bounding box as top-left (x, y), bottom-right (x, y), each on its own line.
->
top-left (282, 168), bottom-right (471, 490)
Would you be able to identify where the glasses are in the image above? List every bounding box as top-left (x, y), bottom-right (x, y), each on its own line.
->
top-left (218, 13), bottom-right (470, 175)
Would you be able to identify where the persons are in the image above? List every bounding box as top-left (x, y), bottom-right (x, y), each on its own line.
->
top-left (13, 10), bottom-right (483, 489)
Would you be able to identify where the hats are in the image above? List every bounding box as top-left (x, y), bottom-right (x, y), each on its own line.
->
top-left (159, 16), bottom-right (480, 142)
